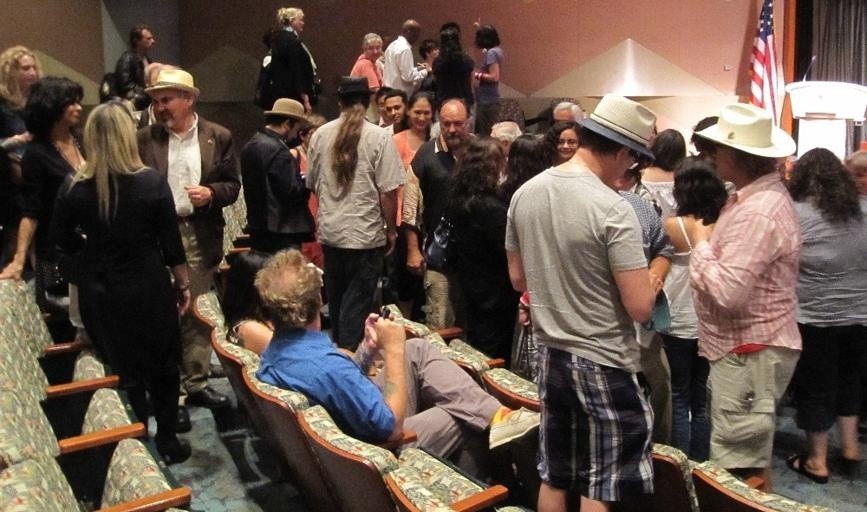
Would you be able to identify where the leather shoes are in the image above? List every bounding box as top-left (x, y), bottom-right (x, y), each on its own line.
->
top-left (188, 386), bottom-right (231, 409)
top-left (174, 406), bottom-right (191, 432)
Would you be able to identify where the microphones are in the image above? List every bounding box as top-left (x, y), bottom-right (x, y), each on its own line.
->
top-left (802, 55), bottom-right (818, 82)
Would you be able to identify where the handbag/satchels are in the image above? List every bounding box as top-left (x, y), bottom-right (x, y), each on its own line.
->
top-left (425, 215), bottom-right (460, 268)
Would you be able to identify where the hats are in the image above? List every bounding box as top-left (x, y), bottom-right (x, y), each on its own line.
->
top-left (330, 76), bottom-right (375, 96)
top-left (573, 93), bottom-right (657, 160)
top-left (144, 68), bottom-right (200, 99)
top-left (263, 98), bottom-right (309, 123)
top-left (694, 104), bottom-right (796, 158)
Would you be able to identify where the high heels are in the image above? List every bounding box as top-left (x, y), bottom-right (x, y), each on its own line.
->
top-left (154, 424), bottom-right (190, 466)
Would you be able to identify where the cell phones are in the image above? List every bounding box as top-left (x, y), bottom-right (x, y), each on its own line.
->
top-left (381, 307), bottom-right (390, 319)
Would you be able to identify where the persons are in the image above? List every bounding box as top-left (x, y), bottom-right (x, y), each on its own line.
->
top-left (61, 101), bottom-right (195, 467)
top-left (249, 245), bottom-right (551, 457)
top-left (842, 147), bottom-right (866, 194)
top-left (787, 145), bottom-right (867, 485)
top-left (0, 7), bottom-right (731, 435)
top-left (690, 101), bottom-right (804, 491)
top-left (505, 90), bottom-right (676, 512)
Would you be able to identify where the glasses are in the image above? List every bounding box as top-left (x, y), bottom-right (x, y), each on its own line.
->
top-left (628, 158), bottom-right (638, 170)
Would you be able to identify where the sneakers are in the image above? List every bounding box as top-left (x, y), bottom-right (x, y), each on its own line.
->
top-left (487, 406), bottom-right (542, 456)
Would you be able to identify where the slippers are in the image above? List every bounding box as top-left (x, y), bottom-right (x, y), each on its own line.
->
top-left (832, 447), bottom-right (863, 464)
top-left (784, 452), bottom-right (828, 483)
